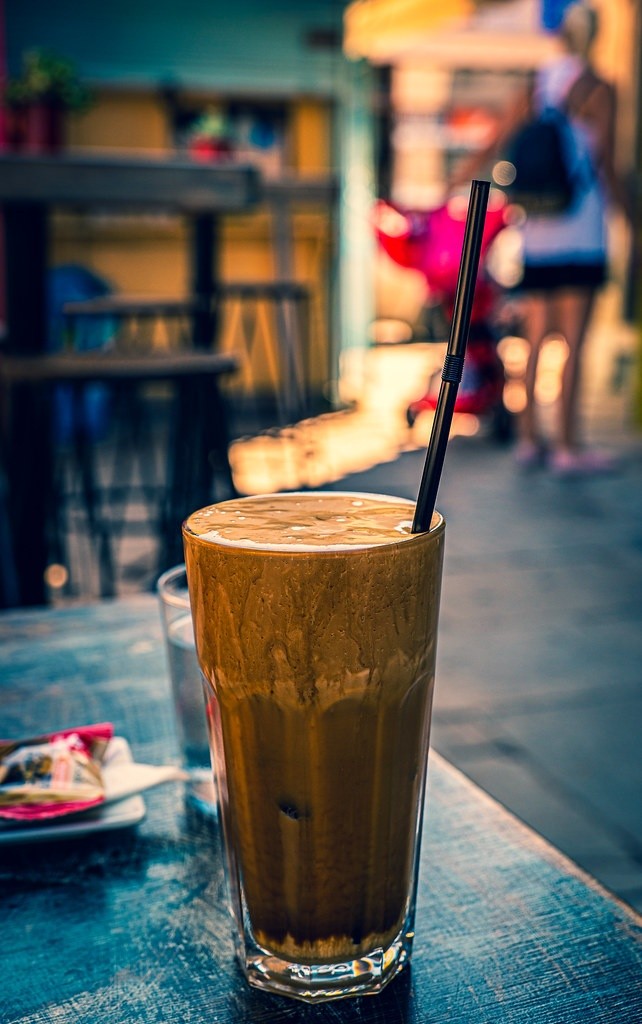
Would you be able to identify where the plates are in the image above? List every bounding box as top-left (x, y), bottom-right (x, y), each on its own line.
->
top-left (0, 732), bottom-right (146, 852)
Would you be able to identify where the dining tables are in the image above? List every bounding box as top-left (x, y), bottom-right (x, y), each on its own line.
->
top-left (2, 593), bottom-right (642, 1023)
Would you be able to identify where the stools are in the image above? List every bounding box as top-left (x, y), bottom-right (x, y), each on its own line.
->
top-left (1, 279), bottom-right (318, 595)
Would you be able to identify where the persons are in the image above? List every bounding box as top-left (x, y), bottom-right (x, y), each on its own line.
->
top-left (443, 3), bottom-right (622, 479)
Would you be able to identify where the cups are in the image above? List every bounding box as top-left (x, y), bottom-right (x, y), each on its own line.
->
top-left (182, 489), bottom-right (446, 1006)
top-left (155, 566), bottom-right (217, 818)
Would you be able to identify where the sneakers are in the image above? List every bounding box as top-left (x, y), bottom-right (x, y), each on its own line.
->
top-left (514, 436), bottom-right (625, 482)
